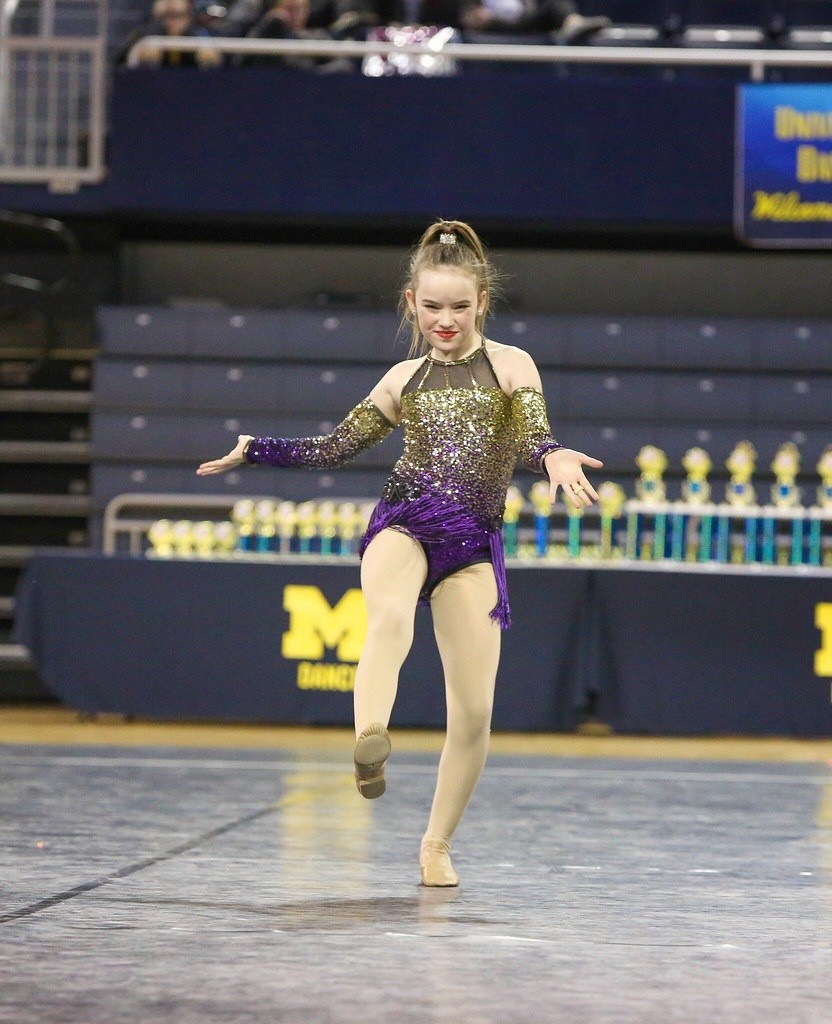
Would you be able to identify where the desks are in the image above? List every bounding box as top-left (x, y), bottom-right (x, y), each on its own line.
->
top-left (6, 557), bottom-right (832, 736)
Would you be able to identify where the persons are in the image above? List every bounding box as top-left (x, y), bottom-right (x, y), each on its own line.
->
top-left (198, 219), bottom-right (603, 887)
top-left (113, 0), bottom-right (610, 69)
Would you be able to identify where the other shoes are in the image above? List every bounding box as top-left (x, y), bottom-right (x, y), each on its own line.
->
top-left (354, 723), bottom-right (390, 800)
top-left (420, 840), bottom-right (460, 887)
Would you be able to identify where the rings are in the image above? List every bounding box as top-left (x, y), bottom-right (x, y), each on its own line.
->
top-left (574, 486), bottom-right (584, 494)
